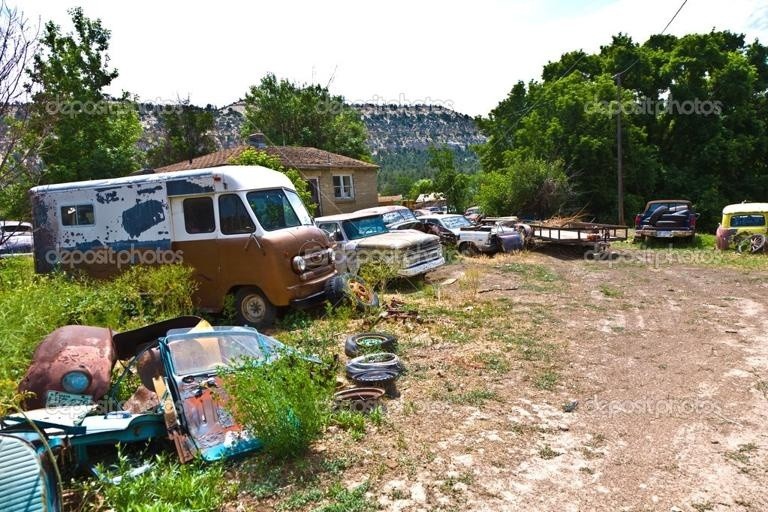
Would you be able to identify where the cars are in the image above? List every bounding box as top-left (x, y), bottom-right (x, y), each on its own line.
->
top-left (308, 204), bottom-right (532, 281)
top-left (716, 201), bottom-right (767, 257)
top-left (0, 219), bottom-right (33, 256)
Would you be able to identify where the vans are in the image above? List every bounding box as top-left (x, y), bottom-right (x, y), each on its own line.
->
top-left (26, 164), bottom-right (339, 327)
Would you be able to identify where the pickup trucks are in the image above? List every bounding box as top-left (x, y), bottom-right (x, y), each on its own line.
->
top-left (633, 199), bottom-right (699, 243)
